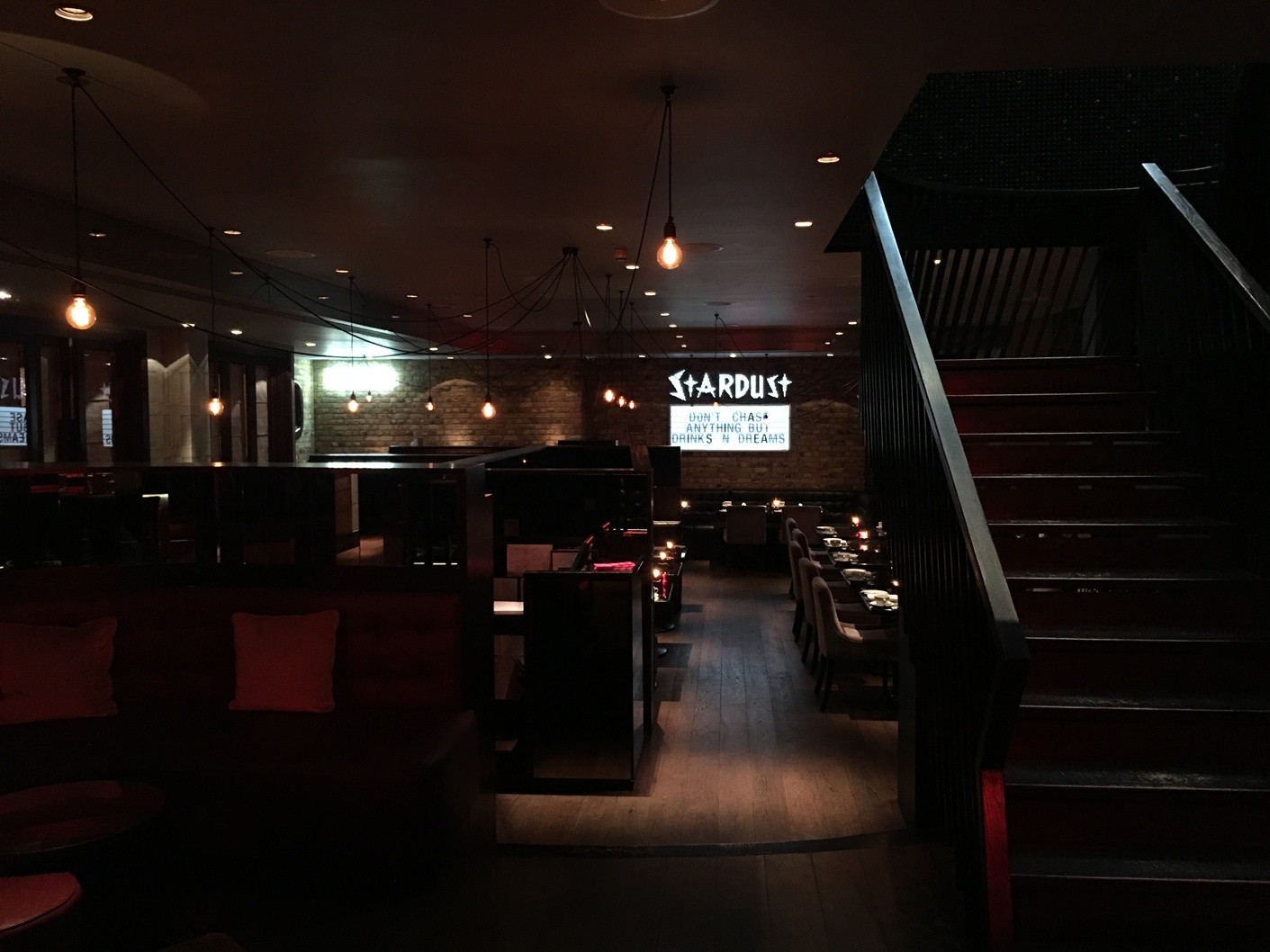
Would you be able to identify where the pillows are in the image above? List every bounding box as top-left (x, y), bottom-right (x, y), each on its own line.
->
top-left (225, 608), bottom-right (340, 715)
top-left (0, 614), bottom-right (119, 728)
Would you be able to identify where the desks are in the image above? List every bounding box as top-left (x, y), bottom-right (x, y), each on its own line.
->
top-left (813, 524), bottom-right (900, 718)
top-left (0, 777), bottom-right (167, 857)
top-left (0, 871), bottom-right (87, 952)
top-left (0, 440), bottom-right (689, 798)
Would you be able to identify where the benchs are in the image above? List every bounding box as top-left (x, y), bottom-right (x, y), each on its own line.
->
top-left (0, 563), bottom-right (483, 886)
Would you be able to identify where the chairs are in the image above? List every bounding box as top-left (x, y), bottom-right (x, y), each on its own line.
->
top-left (785, 517), bottom-right (833, 596)
top-left (810, 576), bottom-right (899, 714)
top-left (799, 556), bottom-right (887, 676)
top-left (789, 528), bottom-right (845, 605)
top-left (778, 503), bottom-right (824, 545)
top-left (788, 539), bottom-right (882, 666)
top-left (723, 502), bottom-right (767, 578)
